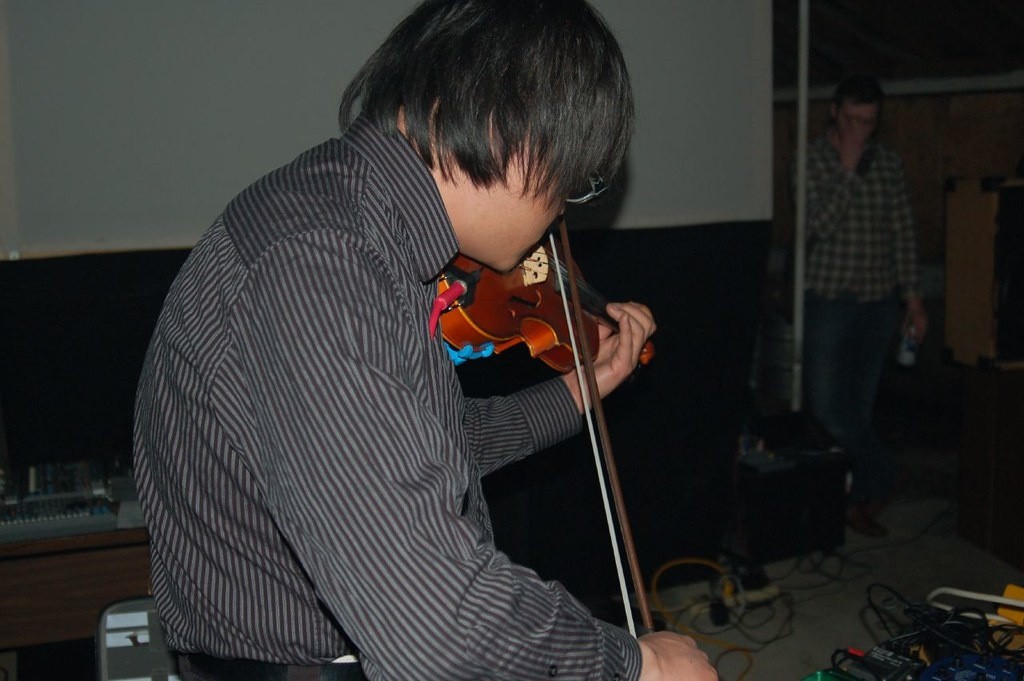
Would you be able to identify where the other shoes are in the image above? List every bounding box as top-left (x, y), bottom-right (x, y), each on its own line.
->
top-left (847, 510), bottom-right (890, 538)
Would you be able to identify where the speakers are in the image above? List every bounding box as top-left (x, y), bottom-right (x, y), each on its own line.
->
top-left (941, 175), bottom-right (1024, 370)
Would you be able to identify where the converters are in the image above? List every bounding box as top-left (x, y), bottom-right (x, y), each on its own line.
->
top-left (861, 646), bottom-right (927, 681)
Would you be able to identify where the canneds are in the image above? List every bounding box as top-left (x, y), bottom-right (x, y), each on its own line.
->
top-left (897, 325), bottom-right (917, 366)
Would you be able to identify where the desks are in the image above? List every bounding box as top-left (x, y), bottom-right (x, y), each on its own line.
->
top-left (0, 528), bottom-right (151, 650)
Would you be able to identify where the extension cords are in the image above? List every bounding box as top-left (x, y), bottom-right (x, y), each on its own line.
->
top-left (693, 583), bottom-right (783, 615)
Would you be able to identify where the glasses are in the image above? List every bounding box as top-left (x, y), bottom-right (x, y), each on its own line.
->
top-left (564, 165), bottom-right (613, 206)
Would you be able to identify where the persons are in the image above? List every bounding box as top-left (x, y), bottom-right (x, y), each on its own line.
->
top-left (132, 0), bottom-right (720, 681)
top-left (776, 67), bottom-right (927, 539)
